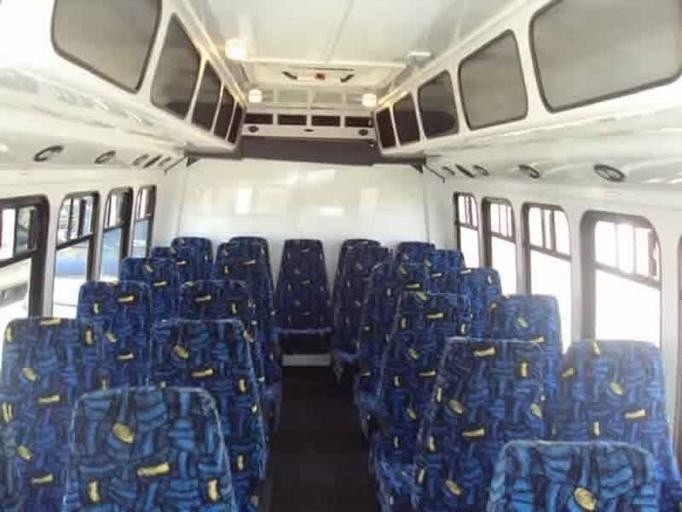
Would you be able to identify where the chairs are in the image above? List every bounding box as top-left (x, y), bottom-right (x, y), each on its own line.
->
top-left (180, 276), bottom-right (282, 449)
top-left (58, 386), bottom-right (237, 511)
top-left (153, 244), bottom-right (204, 287)
top-left (411, 339), bottom-right (543, 512)
top-left (360, 291), bottom-right (472, 447)
top-left (1, 317), bottom-right (121, 512)
top-left (177, 238), bottom-right (441, 341)
top-left (449, 267), bottom-right (502, 338)
top-left (349, 262), bottom-right (432, 426)
top-left (548, 339), bottom-right (682, 512)
top-left (424, 249), bottom-right (468, 293)
top-left (151, 319), bottom-right (267, 508)
top-left (205, 241), bottom-right (281, 369)
top-left (487, 292), bottom-right (561, 413)
top-left (120, 257), bottom-right (179, 326)
top-left (329, 246), bottom-right (409, 390)
top-left (74, 279), bottom-right (153, 388)
top-left (364, 313), bottom-right (483, 512)
top-left (485, 437), bottom-right (662, 512)
